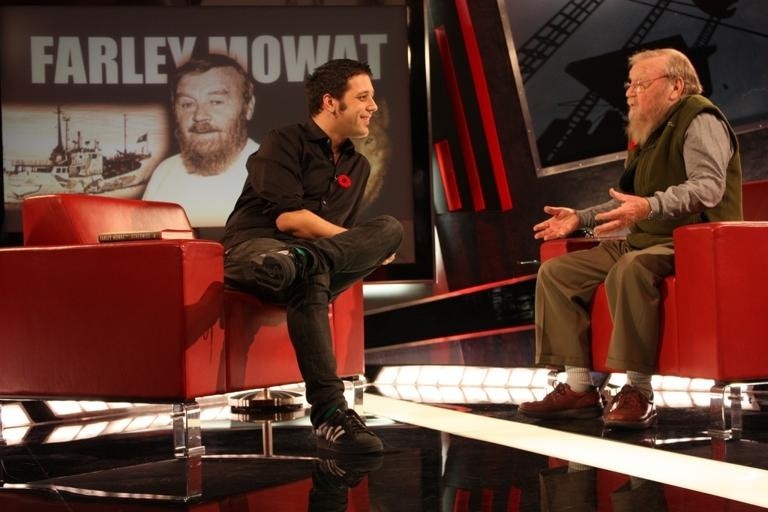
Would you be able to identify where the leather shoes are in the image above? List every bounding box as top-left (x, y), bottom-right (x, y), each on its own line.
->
top-left (603, 383), bottom-right (660, 431)
top-left (517, 381), bottom-right (604, 420)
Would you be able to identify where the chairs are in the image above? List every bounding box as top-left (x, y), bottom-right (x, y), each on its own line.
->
top-left (538, 177), bottom-right (768, 396)
top-left (0, 192), bottom-right (367, 461)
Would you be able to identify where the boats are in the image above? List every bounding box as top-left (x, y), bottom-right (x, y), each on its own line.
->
top-left (3, 104), bottom-right (158, 203)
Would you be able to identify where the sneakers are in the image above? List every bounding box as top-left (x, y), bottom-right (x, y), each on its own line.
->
top-left (312, 406), bottom-right (385, 457)
top-left (222, 248), bottom-right (299, 292)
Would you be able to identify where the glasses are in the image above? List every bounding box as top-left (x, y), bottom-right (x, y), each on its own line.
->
top-left (622, 75), bottom-right (669, 93)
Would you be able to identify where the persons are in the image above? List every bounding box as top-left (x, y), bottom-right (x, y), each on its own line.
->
top-left (219, 58), bottom-right (404, 451)
top-left (538, 461), bottom-right (667, 512)
top-left (140, 53), bottom-right (260, 228)
top-left (516, 49), bottom-right (744, 432)
top-left (299, 454), bottom-right (385, 512)
